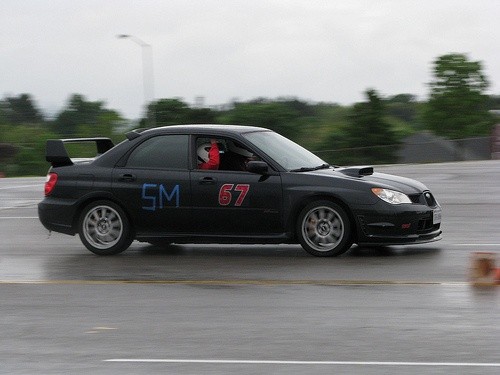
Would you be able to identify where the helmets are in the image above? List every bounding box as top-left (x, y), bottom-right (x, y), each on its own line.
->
top-left (227, 139), bottom-right (253, 157)
top-left (196, 143), bottom-right (212, 162)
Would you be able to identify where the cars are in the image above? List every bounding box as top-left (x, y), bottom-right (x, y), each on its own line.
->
top-left (36, 123), bottom-right (443, 255)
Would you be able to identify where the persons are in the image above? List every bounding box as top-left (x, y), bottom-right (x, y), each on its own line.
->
top-left (196, 136), bottom-right (261, 171)
